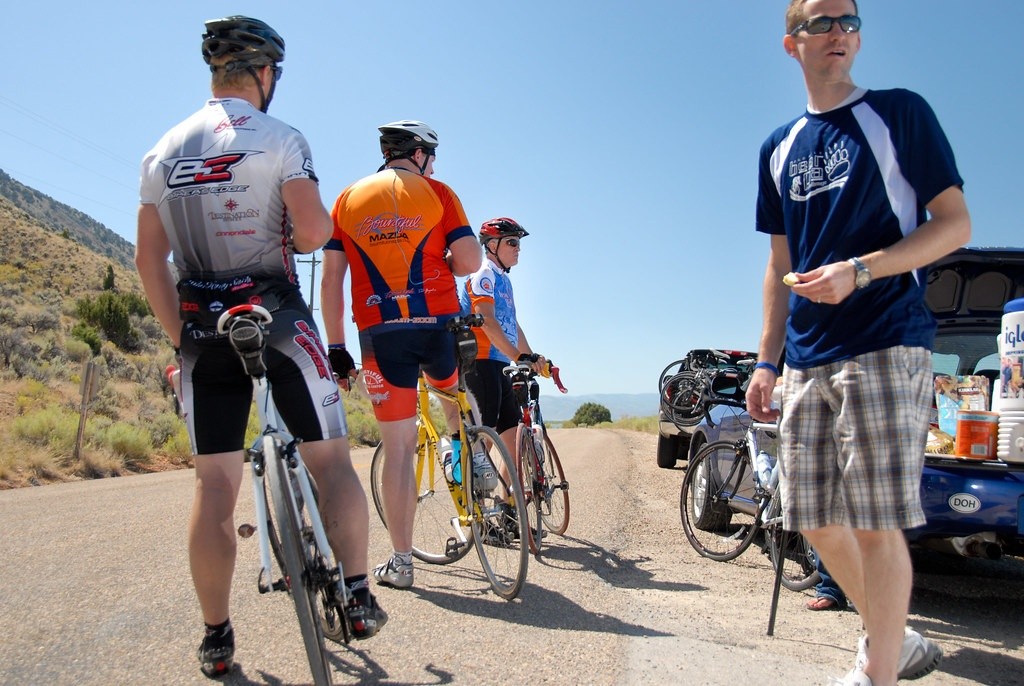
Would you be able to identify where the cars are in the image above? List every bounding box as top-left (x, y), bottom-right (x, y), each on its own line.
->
top-left (657, 346), bottom-right (759, 470)
top-left (687, 242), bottom-right (1024, 601)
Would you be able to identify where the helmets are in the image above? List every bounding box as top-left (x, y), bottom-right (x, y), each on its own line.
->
top-left (479, 217), bottom-right (530, 246)
top-left (377, 119), bottom-right (439, 153)
top-left (200, 14), bottom-right (285, 63)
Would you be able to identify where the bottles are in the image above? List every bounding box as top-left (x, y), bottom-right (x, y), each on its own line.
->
top-left (531, 422), bottom-right (544, 450)
top-left (452, 430), bottom-right (460, 483)
top-left (435, 436), bottom-right (455, 482)
top-left (756, 450), bottom-right (772, 483)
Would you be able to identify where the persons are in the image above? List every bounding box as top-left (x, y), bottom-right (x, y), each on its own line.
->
top-left (135, 15), bottom-right (388, 672)
top-left (322, 119), bottom-right (497, 589)
top-left (746, 0), bottom-right (968, 685)
top-left (459, 217), bottom-right (548, 548)
top-left (809, 549), bottom-right (845, 610)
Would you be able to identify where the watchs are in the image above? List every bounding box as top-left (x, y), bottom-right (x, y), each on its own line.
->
top-left (848, 257), bottom-right (870, 288)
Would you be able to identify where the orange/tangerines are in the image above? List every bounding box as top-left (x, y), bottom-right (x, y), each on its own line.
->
top-left (541, 363), bottom-right (549, 378)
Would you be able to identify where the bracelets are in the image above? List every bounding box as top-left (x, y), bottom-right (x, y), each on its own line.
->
top-left (328, 344), bottom-right (345, 347)
top-left (756, 362), bottom-right (779, 376)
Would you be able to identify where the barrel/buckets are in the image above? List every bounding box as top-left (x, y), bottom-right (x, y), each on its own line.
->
top-left (997, 298), bottom-right (1024, 412)
top-left (955, 409), bottom-right (1001, 459)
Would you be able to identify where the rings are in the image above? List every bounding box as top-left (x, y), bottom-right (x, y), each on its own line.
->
top-left (818, 297), bottom-right (821, 303)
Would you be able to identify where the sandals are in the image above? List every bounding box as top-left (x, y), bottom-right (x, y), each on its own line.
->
top-left (807, 596), bottom-right (833, 611)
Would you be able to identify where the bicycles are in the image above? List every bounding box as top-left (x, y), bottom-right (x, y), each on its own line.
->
top-left (678, 386), bottom-right (822, 594)
top-left (501, 365), bottom-right (569, 554)
top-left (347, 314), bottom-right (530, 601)
top-left (162, 303), bottom-right (380, 686)
top-left (658, 351), bottom-right (760, 437)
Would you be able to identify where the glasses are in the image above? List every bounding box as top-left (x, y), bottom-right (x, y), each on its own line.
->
top-left (269, 65), bottom-right (283, 82)
top-left (792, 14), bottom-right (861, 37)
top-left (497, 239), bottom-right (520, 248)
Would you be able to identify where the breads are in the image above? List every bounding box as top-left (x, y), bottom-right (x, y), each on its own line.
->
top-left (782, 272), bottom-right (800, 286)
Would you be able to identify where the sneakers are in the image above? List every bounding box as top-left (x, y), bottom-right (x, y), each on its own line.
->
top-left (826, 668), bottom-right (871, 686)
top-left (474, 459), bottom-right (498, 492)
top-left (503, 518), bottom-right (547, 540)
top-left (479, 522), bottom-right (510, 548)
top-left (347, 595), bottom-right (388, 642)
top-left (855, 626), bottom-right (942, 680)
top-left (196, 643), bottom-right (234, 675)
top-left (371, 561), bottom-right (414, 588)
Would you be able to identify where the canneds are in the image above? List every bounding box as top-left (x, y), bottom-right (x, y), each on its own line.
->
top-left (954, 410), bottom-right (1000, 461)
